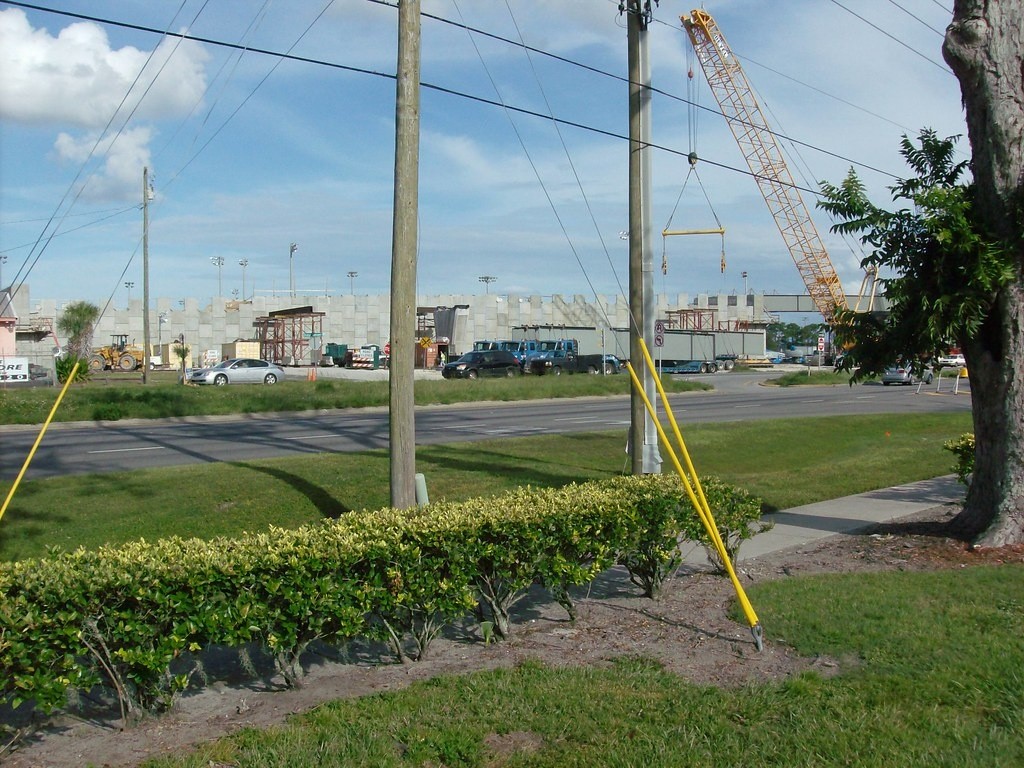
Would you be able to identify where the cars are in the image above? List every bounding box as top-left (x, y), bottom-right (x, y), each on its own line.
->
top-left (939, 353), bottom-right (968, 367)
top-left (880, 359), bottom-right (935, 385)
top-left (190, 358), bottom-right (286, 386)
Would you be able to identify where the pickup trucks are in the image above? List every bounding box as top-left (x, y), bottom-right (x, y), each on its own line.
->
top-left (531, 348), bottom-right (604, 377)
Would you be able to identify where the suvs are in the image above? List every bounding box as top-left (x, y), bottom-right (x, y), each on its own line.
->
top-left (441, 350), bottom-right (522, 381)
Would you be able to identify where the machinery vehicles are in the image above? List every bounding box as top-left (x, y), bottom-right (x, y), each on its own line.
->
top-left (88, 334), bottom-right (145, 372)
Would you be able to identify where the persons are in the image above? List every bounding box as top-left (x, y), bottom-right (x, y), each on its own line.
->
top-left (957, 364), bottom-right (968, 378)
top-left (439, 351), bottom-right (446, 366)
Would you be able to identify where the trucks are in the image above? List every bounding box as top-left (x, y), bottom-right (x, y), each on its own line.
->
top-left (143, 356), bottom-right (163, 371)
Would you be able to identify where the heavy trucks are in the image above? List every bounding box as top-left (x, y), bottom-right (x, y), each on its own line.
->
top-left (473, 339), bottom-right (740, 375)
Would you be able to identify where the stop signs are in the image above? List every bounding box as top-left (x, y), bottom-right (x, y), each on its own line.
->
top-left (384, 344), bottom-right (390, 354)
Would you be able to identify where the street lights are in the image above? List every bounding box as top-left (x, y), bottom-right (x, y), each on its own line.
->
top-left (347, 272), bottom-right (359, 294)
top-left (238, 259), bottom-right (248, 303)
top-left (289, 242), bottom-right (299, 300)
top-left (210, 257), bottom-right (226, 300)
top-left (478, 276), bottom-right (499, 294)
top-left (124, 281), bottom-right (135, 308)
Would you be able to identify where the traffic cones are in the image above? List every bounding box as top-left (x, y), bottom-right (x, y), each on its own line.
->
top-left (310, 367), bottom-right (316, 382)
top-left (307, 367), bottom-right (312, 380)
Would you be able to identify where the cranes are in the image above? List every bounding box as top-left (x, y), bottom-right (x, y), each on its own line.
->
top-left (659, 7), bottom-right (884, 359)
